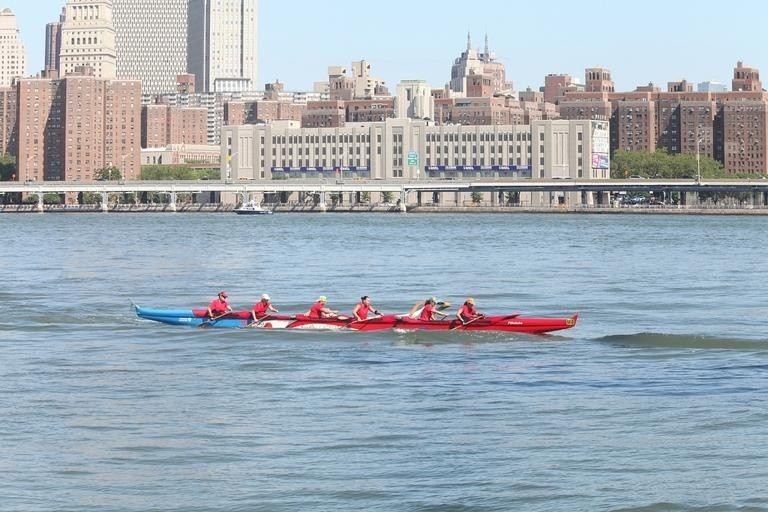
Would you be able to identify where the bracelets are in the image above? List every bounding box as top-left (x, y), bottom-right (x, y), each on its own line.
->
top-left (375, 310), bottom-right (377, 314)
top-left (461, 319), bottom-right (464, 322)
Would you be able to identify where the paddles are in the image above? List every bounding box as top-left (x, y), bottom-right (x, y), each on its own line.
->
top-left (340, 316), bottom-right (382, 329)
top-left (198, 313), bottom-right (231, 327)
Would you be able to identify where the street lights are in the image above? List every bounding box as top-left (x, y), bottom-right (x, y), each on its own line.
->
top-left (27, 154), bottom-right (38, 184)
top-left (341, 151), bottom-right (350, 183)
top-left (229, 151), bottom-right (239, 180)
top-left (697, 138), bottom-right (705, 185)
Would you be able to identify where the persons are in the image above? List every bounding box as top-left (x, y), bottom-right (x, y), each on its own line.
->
top-left (251, 293), bottom-right (279, 323)
top-left (409, 295), bottom-right (452, 321)
top-left (207, 290), bottom-right (232, 318)
top-left (309, 296), bottom-right (339, 319)
top-left (456, 296), bottom-right (485, 326)
top-left (352, 295), bottom-right (384, 322)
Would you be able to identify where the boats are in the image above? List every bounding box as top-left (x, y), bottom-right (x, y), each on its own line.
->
top-left (129, 304), bottom-right (579, 334)
top-left (232, 202), bottom-right (273, 215)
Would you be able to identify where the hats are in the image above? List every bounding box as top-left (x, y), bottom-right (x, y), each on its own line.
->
top-left (466, 297), bottom-right (475, 305)
top-left (316, 295), bottom-right (327, 302)
top-left (262, 293), bottom-right (270, 300)
top-left (219, 291), bottom-right (229, 298)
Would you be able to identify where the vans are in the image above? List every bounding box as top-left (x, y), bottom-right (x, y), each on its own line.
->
top-left (629, 175), bottom-right (645, 180)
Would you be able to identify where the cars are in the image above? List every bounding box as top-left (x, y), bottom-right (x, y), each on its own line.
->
top-left (352, 177), bottom-right (369, 182)
